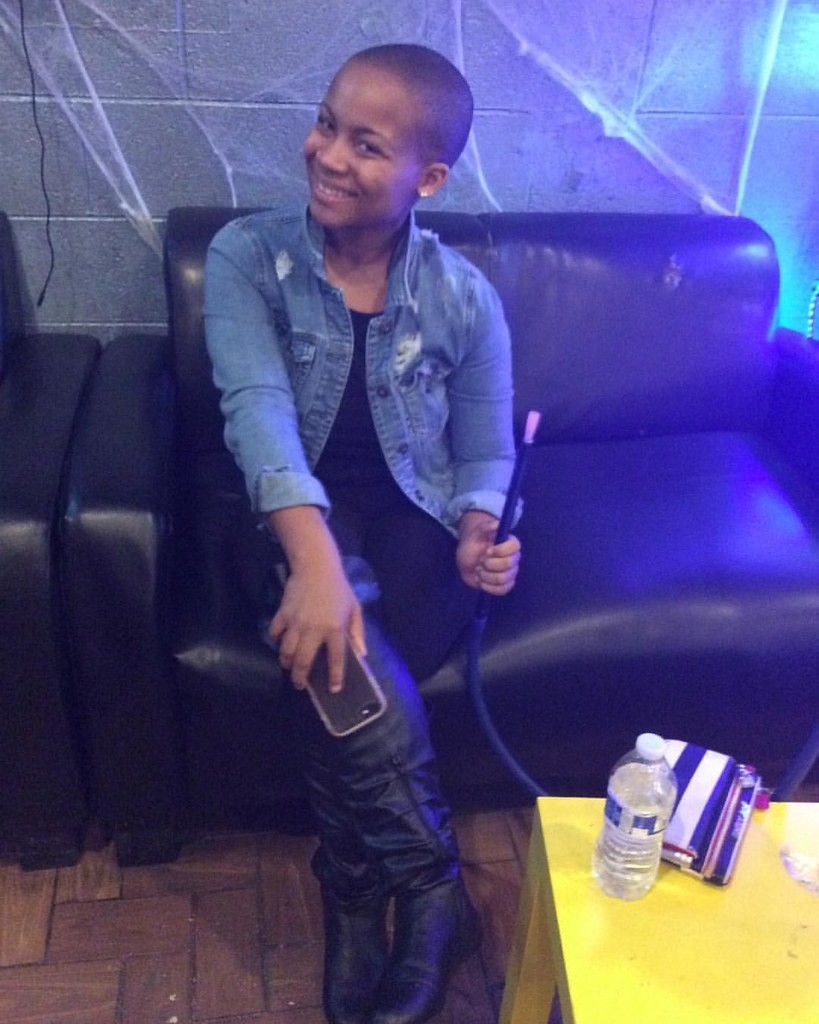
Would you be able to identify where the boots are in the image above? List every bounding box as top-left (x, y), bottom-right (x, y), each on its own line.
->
top-left (276, 554), bottom-right (484, 1023)
top-left (275, 666), bottom-right (394, 1022)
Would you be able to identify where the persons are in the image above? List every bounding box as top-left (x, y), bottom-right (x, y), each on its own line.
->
top-left (201, 44), bottom-right (523, 1024)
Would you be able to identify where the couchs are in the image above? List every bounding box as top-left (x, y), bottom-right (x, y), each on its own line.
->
top-left (1, 197), bottom-right (819, 878)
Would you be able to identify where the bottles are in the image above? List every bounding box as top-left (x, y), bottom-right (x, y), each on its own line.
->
top-left (593, 732), bottom-right (679, 902)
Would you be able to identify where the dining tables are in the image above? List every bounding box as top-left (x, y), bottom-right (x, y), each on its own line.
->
top-left (494, 795), bottom-right (819, 1024)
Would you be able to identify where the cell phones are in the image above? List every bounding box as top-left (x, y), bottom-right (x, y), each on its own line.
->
top-left (304, 633), bottom-right (387, 739)
